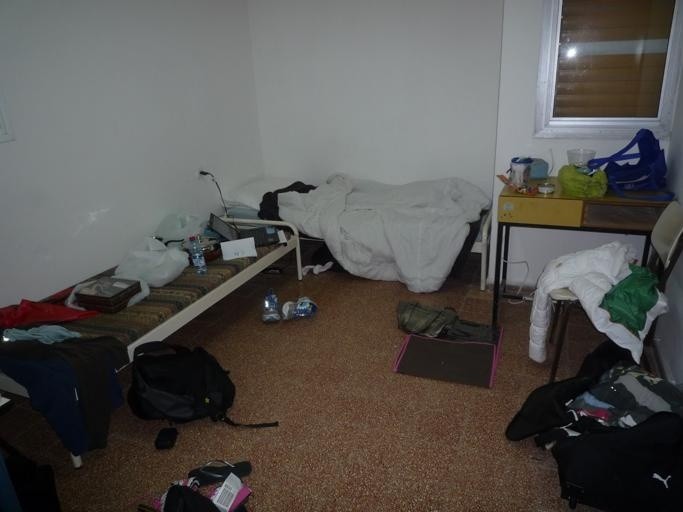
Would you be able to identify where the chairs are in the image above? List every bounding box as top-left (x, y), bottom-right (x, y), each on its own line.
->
top-left (548, 199), bottom-right (682, 383)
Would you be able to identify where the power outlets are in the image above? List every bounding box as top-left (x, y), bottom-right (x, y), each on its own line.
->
top-left (195, 169), bottom-right (202, 179)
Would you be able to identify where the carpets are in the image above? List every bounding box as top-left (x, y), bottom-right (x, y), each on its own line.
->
top-left (393, 323), bottom-right (504, 389)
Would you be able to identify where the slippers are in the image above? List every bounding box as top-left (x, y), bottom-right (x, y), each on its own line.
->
top-left (187, 459), bottom-right (252, 487)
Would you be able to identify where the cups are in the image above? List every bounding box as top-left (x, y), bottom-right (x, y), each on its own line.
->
top-left (567, 148), bottom-right (595, 172)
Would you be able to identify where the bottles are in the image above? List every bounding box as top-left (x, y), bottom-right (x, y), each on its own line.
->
top-left (188, 236), bottom-right (208, 276)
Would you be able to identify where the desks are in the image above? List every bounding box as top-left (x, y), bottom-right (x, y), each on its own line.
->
top-left (491, 176), bottom-right (677, 323)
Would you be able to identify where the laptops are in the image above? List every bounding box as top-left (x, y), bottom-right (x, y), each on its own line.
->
top-left (207, 212), bottom-right (280, 247)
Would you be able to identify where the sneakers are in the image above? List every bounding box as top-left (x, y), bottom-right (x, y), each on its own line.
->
top-left (261, 287), bottom-right (282, 324)
top-left (281, 296), bottom-right (318, 320)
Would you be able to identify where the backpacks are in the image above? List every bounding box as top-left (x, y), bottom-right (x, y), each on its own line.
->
top-left (127, 341), bottom-right (279, 430)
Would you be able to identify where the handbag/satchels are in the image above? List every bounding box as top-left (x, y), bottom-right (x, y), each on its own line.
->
top-left (505, 375), bottom-right (599, 441)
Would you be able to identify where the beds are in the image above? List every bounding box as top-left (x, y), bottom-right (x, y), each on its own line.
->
top-left (223, 208), bottom-right (492, 292)
top-left (0, 218), bottom-right (302, 469)
